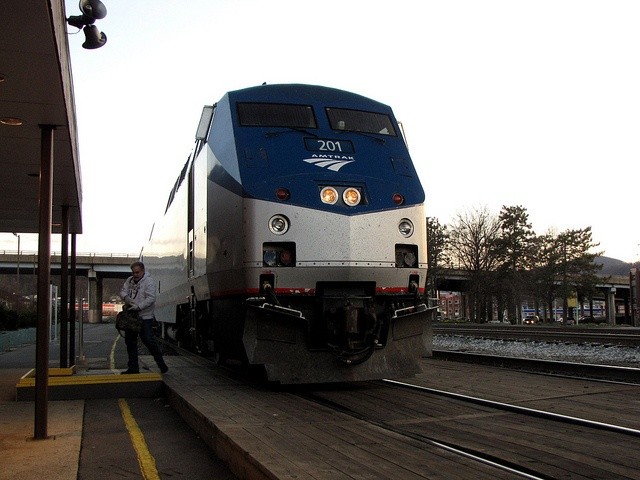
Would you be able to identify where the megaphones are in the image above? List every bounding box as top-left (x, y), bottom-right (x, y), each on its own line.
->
top-left (79, 0), bottom-right (107, 24)
top-left (81, 25), bottom-right (107, 50)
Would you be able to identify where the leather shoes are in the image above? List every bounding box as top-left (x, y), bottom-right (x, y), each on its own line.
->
top-left (160, 365), bottom-right (168, 374)
top-left (120, 369), bottom-right (139, 374)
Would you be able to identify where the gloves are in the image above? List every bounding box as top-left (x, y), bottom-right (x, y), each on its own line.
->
top-left (127, 303), bottom-right (139, 312)
top-left (125, 296), bottom-right (133, 306)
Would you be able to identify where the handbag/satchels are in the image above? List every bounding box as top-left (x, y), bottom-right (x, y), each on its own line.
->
top-left (115, 305), bottom-right (142, 332)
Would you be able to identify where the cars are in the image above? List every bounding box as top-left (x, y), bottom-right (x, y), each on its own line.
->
top-left (579, 316), bottom-right (593, 320)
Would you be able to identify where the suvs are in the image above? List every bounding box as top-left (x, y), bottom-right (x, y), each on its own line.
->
top-left (522, 315), bottom-right (540, 324)
top-left (557, 317), bottom-right (576, 325)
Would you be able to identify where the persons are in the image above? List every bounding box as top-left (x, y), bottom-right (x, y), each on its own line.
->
top-left (119, 262), bottom-right (168, 375)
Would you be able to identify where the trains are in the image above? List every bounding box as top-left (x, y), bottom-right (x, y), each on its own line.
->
top-left (138, 82), bottom-right (439, 386)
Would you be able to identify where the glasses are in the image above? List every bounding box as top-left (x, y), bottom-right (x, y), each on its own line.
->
top-left (131, 272), bottom-right (139, 275)
